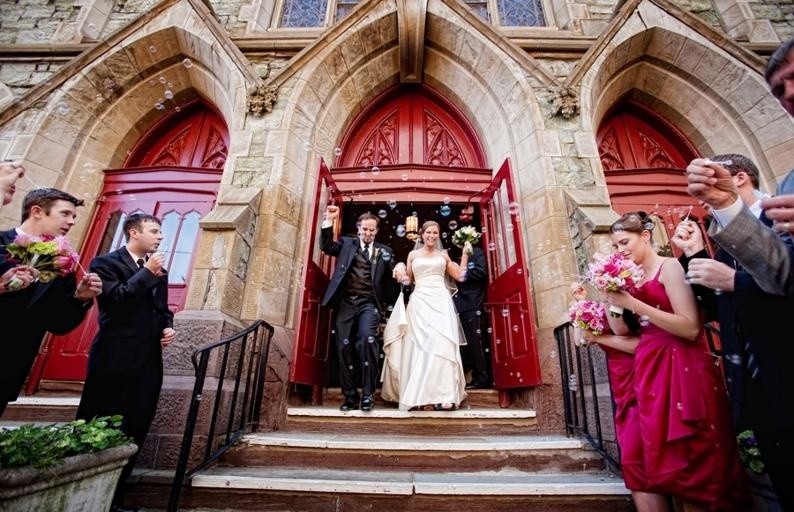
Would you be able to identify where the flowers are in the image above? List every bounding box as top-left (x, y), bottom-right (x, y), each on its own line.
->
top-left (10, 233), bottom-right (78, 290)
top-left (452, 225), bottom-right (480, 256)
top-left (561, 284), bottom-right (609, 344)
top-left (588, 255), bottom-right (645, 316)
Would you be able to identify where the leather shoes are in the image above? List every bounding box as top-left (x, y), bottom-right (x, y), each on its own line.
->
top-left (361, 394), bottom-right (374, 412)
top-left (464, 380), bottom-right (492, 390)
top-left (339, 400), bottom-right (360, 410)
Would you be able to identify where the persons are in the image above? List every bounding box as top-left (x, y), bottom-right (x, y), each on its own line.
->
top-left (570, 283), bottom-right (641, 512)
top-left (452, 248), bottom-right (492, 389)
top-left (1, 188), bottom-right (103, 420)
top-left (75, 214), bottom-right (176, 509)
top-left (686, 38), bottom-right (794, 296)
top-left (671, 155), bottom-right (793, 511)
top-left (318, 206), bottom-right (405, 411)
top-left (0, 161), bottom-right (25, 218)
top-left (603, 211), bottom-right (743, 512)
top-left (379, 219), bottom-right (473, 410)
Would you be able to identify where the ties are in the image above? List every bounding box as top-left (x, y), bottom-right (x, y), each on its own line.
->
top-left (733, 257), bottom-right (759, 381)
top-left (137, 258), bottom-right (145, 270)
top-left (363, 243), bottom-right (370, 259)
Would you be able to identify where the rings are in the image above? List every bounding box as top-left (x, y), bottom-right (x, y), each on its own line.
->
top-left (783, 222), bottom-right (790, 233)
